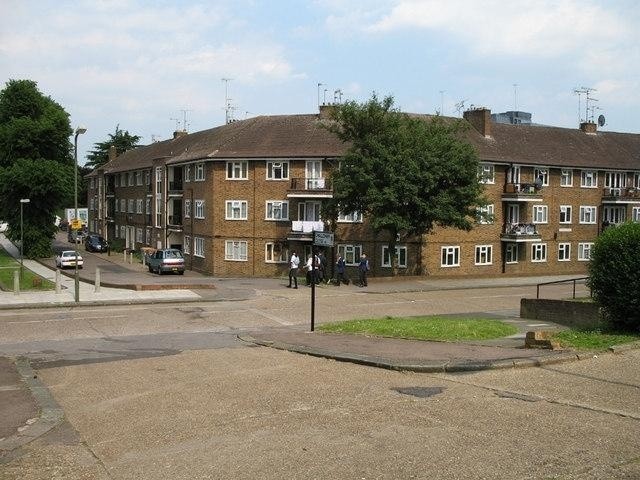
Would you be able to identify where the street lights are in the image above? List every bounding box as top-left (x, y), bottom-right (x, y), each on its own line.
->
top-left (20, 199), bottom-right (30, 280)
top-left (73, 125), bottom-right (88, 302)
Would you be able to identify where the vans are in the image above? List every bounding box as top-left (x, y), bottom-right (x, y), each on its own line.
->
top-left (148, 248), bottom-right (186, 276)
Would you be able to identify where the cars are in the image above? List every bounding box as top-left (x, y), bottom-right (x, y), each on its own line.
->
top-left (55, 250), bottom-right (84, 269)
top-left (67, 230), bottom-right (86, 244)
top-left (85, 234), bottom-right (108, 253)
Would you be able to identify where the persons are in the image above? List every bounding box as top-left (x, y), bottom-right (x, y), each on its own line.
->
top-left (286, 250), bottom-right (300, 289)
top-left (303, 253), bottom-right (312, 287)
top-left (333, 253), bottom-right (352, 286)
top-left (313, 251), bottom-right (321, 284)
top-left (357, 253), bottom-right (371, 287)
top-left (318, 251), bottom-right (331, 285)
